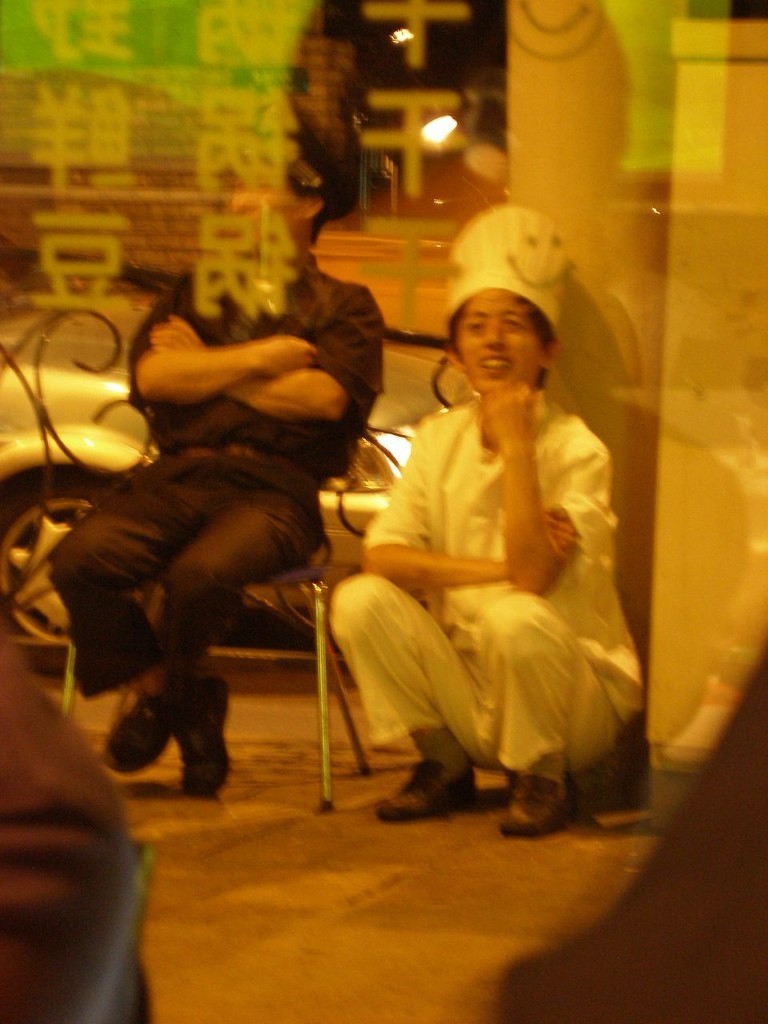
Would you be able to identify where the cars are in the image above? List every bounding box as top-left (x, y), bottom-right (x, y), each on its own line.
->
top-left (0, 363), bottom-right (419, 679)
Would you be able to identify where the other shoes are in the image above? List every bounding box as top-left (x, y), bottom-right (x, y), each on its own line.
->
top-left (499, 775), bottom-right (572, 837)
top-left (148, 674), bottom-right (230, 798)
top-left (375, 759), bottom-right (480, 821)
top-left (105, 691), bottom-right (173, 772)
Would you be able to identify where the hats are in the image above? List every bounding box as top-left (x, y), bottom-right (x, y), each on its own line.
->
top-left (286, 120), bottom-right (359, 242)
top-left (442, 205), bottom-right (567, 341)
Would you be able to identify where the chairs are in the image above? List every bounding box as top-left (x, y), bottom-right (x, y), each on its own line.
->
top-left (62, 567), bottom-right (336, 814)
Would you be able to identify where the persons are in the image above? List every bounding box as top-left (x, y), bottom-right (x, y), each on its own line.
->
top-left (330, 208), bottom-right (645, 834)
top-left (46, 126), bottom-right (388, 801)
